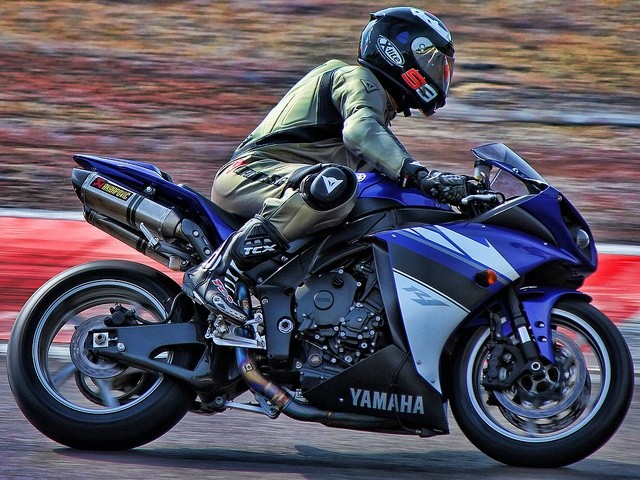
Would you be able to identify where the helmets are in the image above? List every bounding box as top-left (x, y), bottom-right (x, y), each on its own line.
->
top-left (357, 5), bottom-right (455, 118)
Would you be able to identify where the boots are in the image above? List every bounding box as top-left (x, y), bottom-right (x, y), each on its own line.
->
top-left (183, 214), bottom-right (290, 325)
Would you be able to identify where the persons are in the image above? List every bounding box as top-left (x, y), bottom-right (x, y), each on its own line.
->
top-left (181, 7), bottom-right (488, 322)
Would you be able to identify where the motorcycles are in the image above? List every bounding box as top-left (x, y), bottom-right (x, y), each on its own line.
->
top-left (5, 143), bottom-right (634, 469)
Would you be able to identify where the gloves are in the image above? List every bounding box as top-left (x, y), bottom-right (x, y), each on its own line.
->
top-left (421, 171), bottom-right (484, 206)
